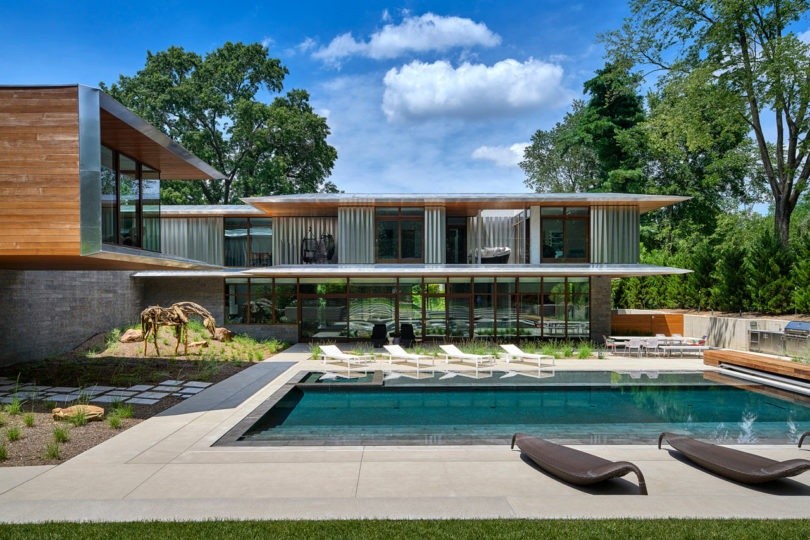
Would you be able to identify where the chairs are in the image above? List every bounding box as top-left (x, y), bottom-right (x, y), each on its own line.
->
top-left (439, 343), bottom-right (496, 368)
top-left (623, 338), bottom-right (641, 358)
top-left (603, 335), bottom-right (617, 353)
top-left (640, 338), bottom-right (659, 358)
top-left (655, 334), bottom-right (668, 345)
top-left (499, 343), bottom-right (556, 368)
top-left (581, 322), bottom-right (590, 334)
top-left (672, 333), bottom-right (682, 337)
top-left (316, 344), bottom-right (367, 367)
top-left (383, 344), bottom-right (435, 368)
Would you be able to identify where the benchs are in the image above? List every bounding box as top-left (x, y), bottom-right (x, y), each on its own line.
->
top-left (658, 345), bottom-right (709, 359)
top-left (469, 250), bottom-right (510, 264)
top-left (546, 327), bottom-right (577, 334)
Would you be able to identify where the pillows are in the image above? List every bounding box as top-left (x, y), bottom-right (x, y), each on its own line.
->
top-left (494, 248), bottom-right (503, 256)
top-left (303, 249), bottom-right (316, 259)
top-left (476, 248), bottom-right (485, 256)
top-left (484, 247), bottom-right (495, 256)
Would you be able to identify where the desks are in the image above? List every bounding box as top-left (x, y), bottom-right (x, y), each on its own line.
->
top-left (544, 320), bottom-right (589, 334)
top-left (609, 335), bottom-right (693, 353)
